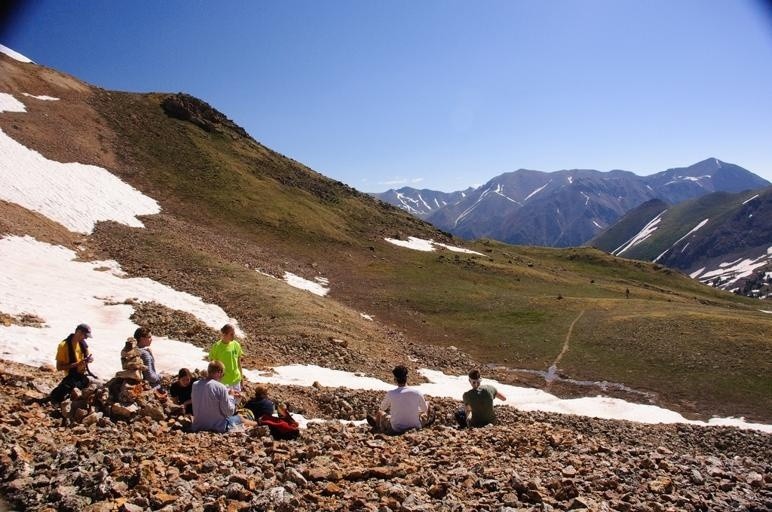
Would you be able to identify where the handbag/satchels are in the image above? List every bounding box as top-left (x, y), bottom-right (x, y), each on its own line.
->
top-left (256, 414), bottom-right (299, 441)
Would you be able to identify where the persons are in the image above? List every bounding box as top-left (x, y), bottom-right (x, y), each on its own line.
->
top-left (454, 370), bottom-right (506, 428)
top-left (367, 364), bottom-right (428, 435)
top-left (190, 359), bottom-right (241, 433)
top-left (208, 324), bottom-right (246, 406)
top-left (135, 327), bottom-right (170, 402)
top-left (56, 323), bottom-right (95, 384)
top-left (240, 385), bottom-right (274, 427)
top-left (170, 368), bottom-right (199, 415)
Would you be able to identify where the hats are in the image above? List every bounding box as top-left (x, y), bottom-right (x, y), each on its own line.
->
top-left (76, 324), bottom-right (93, 338)
